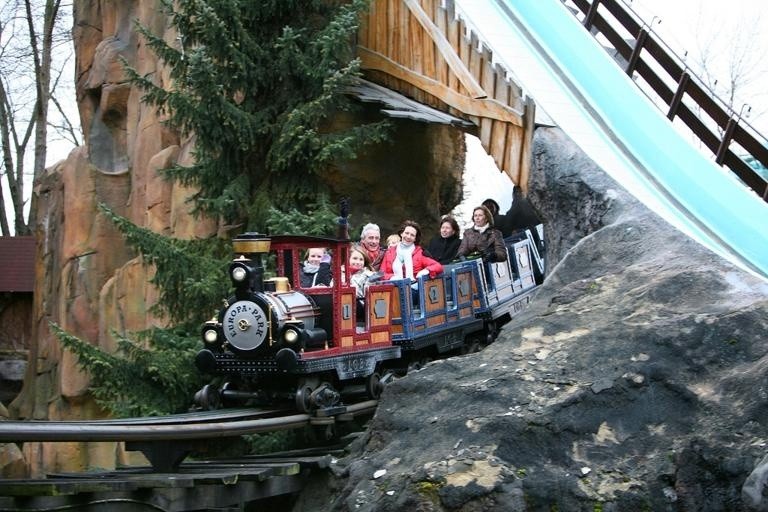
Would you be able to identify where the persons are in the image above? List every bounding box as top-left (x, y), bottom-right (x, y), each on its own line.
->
top-left (387, 236), bottom-right (401, 250)
top-left (299, 246), bottom-right (332, 288)
top-left (361, 225), bottom-right (385, 268)
top-left (482, 201), bottom-right (512, 240)
top-left (430, 218), bottom-right (463, 261)
top-left (381, 222), bottom-right (443, 302)
top-left (457, 208), bottom-right (507, 264)
top-left (332, 251), bottom-right (380, 321)
